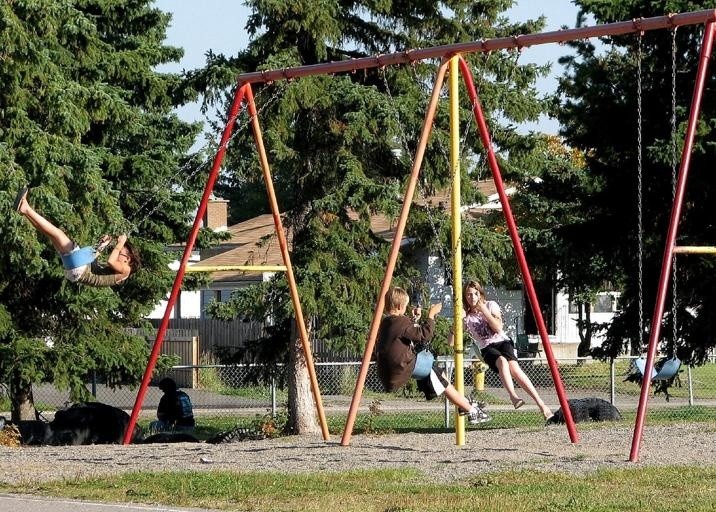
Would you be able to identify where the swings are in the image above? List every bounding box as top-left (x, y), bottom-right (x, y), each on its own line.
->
top-left (60, 79), bottom-right (289, 269)
top-left (634, 26), bottom-right (681, 380)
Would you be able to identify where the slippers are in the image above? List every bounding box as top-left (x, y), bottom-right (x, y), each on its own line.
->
top-left (11, 186), bottom-right (30, 214)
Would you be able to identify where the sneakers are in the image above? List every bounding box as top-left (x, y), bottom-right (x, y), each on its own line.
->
top-left (466, 407), bottom-right (494, 424)
top-left (457, 398), bottom-right (489, 417)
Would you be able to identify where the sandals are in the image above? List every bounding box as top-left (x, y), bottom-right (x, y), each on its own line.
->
top-left (511, 398), bottom-right (526, 410)
top-left (543, 409), bottom-right (556, 422)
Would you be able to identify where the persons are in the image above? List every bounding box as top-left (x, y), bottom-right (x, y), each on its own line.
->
top-left (13, 188), bottom-right (141, 287)
top-left (376, 286), bottom-right (493, 424)
top-left (448, 281), bottom-right (554, 420)
top-left (150, 378), bottom-right (195, 431)
